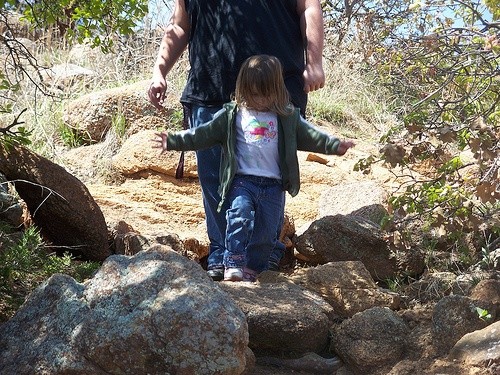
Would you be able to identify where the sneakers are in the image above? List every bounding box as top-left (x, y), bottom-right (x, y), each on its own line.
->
top-left (225, 267), bottom-right (244, 283)
top-left (206, 263), bottom-right (222, 280)
top-left (241, 269), bottom-right (257, 283)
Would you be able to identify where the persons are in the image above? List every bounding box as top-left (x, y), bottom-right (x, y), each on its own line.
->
top-left (151, 54), bottom-right (358, 279)
top-left (148, 0), bottom-right (325, 280)
